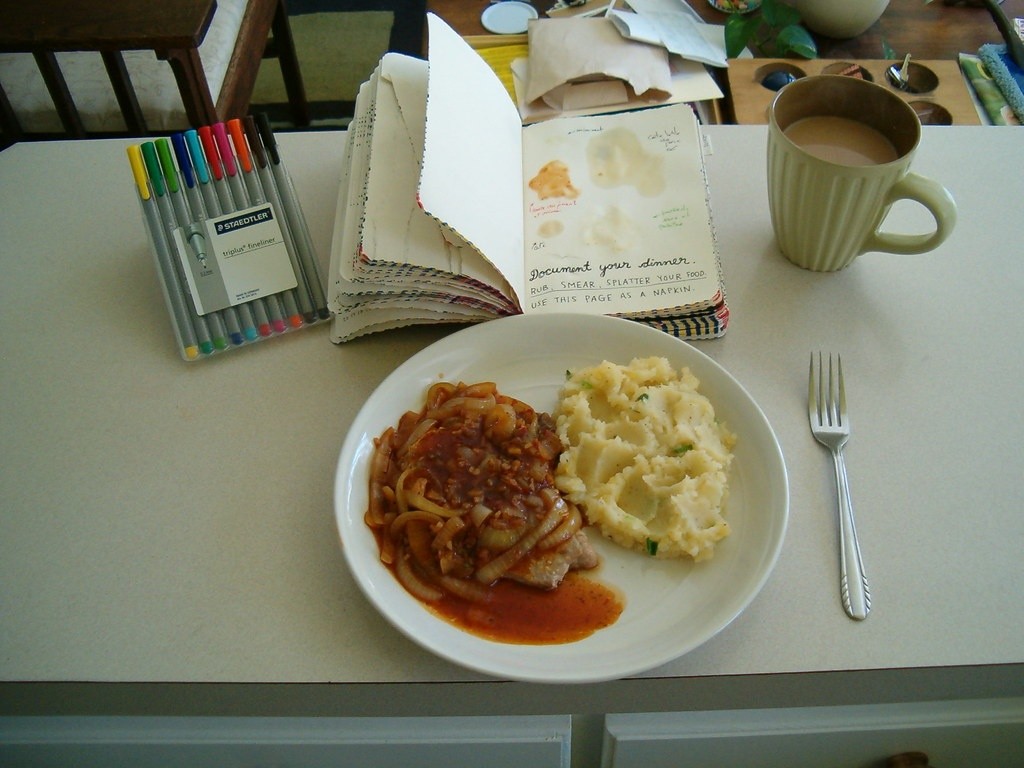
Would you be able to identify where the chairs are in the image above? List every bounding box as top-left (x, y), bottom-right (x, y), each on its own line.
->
top-left (0, 0), bottom-right (312, 149)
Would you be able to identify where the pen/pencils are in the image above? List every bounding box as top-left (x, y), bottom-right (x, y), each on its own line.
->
top-left (126, 115), bottom-right (334, 360)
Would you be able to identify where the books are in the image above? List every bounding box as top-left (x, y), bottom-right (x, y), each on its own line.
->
top-left (324, 11), bottom-right (727, 346)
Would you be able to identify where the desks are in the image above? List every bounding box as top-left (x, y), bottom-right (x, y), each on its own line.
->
top-left (0, 123), bottom-right (1024, 768)
top-left (423, 0), bottom-right (1024, 123)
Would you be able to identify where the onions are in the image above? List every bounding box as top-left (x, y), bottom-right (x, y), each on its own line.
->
top-left (364, 382), bottom-right (584, 601)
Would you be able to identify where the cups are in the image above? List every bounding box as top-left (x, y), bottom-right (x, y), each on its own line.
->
top-left (765, 74), bottom-right (959, 274)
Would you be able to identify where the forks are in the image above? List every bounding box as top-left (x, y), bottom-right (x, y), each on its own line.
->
top-left (808, 350), bottom-right (873, 621)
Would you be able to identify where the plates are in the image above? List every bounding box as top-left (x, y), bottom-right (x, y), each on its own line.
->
top-left (332, 312), bottom-right (791, 685)
